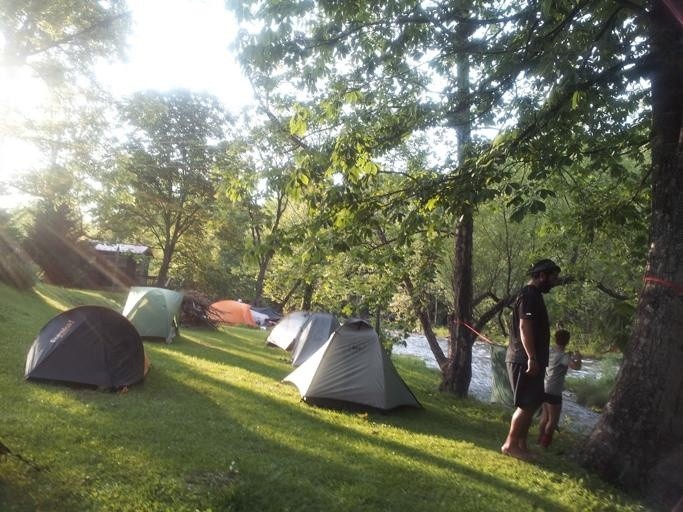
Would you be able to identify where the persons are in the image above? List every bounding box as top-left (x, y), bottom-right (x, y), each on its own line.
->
top-left (533, 330), bottom-right (582, 451)
top-left (499, 258), bottom-right (561, 464)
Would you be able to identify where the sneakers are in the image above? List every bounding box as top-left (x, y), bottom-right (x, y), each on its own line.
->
top-left (501, 439), bottom-right (549, 463)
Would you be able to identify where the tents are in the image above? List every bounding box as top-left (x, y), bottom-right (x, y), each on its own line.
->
top-left (248, 304), bottom-right (284, 328)
top-left (203, 299), bottom-right (255, 326)
top-left (281, 317), bottom-right (424, 412)
top-left (123, 285), bottom-right (184, 341)
top-left (22, 305), bottom-right (150, 388)
top-left (266, 311), bottom-right (313, 350)
top-left (286, 312), bottom-right (339, 367)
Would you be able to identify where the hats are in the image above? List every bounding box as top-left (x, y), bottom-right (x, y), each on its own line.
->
top-left (526, 259), bottom-right (561, 275)
